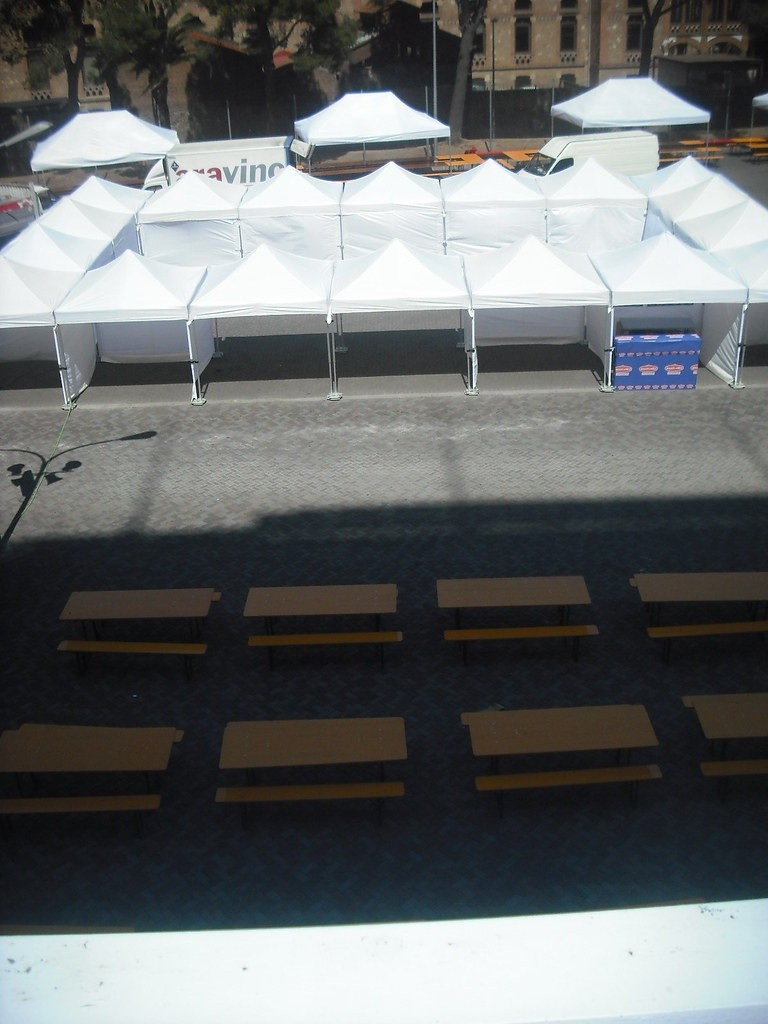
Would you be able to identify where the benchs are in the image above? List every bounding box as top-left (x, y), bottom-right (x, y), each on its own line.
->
top-left (212, 591), bottom-right (222, 601)
top-left (682, 695), bottom-right (695, 708)
top-left (19, 723), bottom-right (184, 743)
top-left (0, 793), bottom-right (162, 839)
top-left (646, 620), bottom-right (768, 664)
top-left (474, 764), bottom-right (664, 822)
top-left (57, 640), bottom-right (207, 680)
top-left (443, 624), bottom-right (600, 669)
top-left (215, 781), bottom-right (406, 830)
top-left (700, 759), bottom-right (768, 804)
top-left (248, 629), bottom-right (403, 676)
top-left (629, 577), bottom-right (651, 613)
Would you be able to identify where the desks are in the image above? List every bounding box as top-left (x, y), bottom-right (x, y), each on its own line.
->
top-left (0, 727), bottom-right (178, 794)
top-left (244, 583), bottom-right (398, 631)
top-left (293, 134), bottom-right (768, 181)
top-left (692, 692), bottom-right (768, 761)
top-left (60, 587), bottom-right (214, 638)
top-left (218, 716), bottom-right (408, 787)
top-left (633, 571), bottom-right (768, 625)
top-left (435, 575), bottom-right (591, 629)
top-left (468, 704), bottom-right (659, 775)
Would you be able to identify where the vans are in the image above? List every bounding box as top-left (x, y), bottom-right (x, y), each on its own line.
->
top-left (518, 131), bottom-right (660, 181)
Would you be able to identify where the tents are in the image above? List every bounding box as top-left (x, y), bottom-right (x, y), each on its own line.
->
top-left (0, 155), bottom-right (768, 411)
top-left (750, 93), bottom-right (768, 138)
top-left (293, 91), bottom-right (452, 177)
top-left (550, 79), bottom-right (711, 169)
top-left (30, 109), bottom-right (180, 188)
